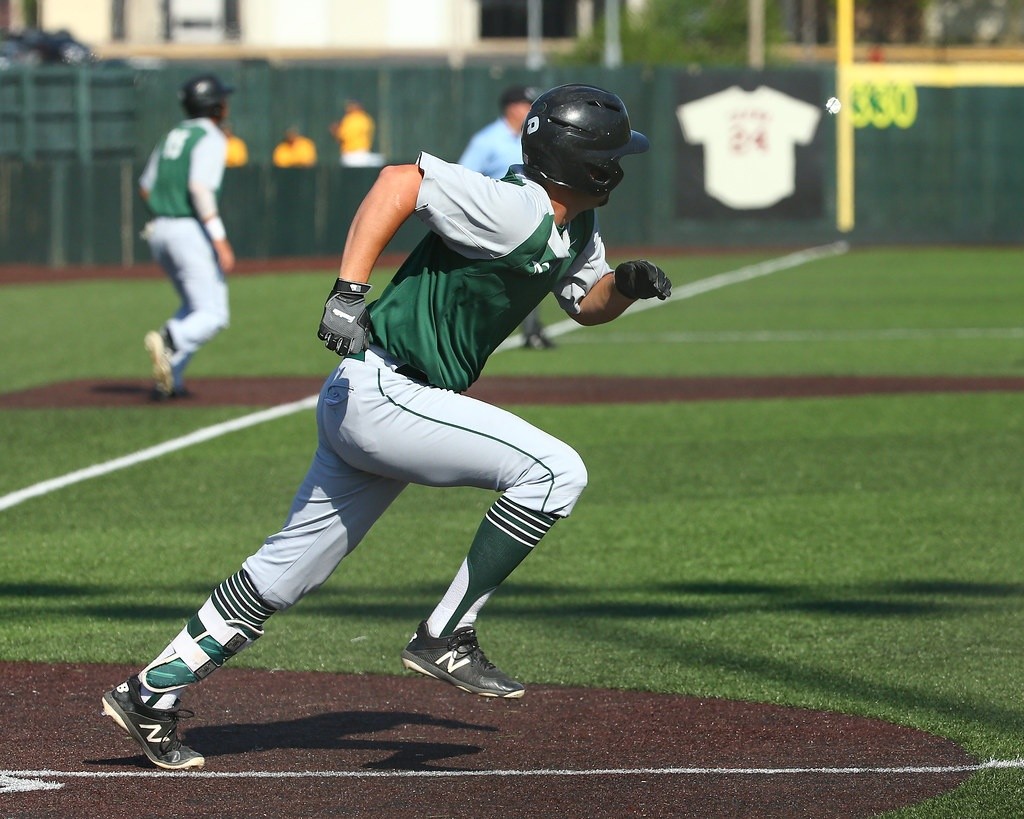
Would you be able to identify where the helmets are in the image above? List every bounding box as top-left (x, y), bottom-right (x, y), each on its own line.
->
top-left (178, 73), bottom-right (234, 120)
top-left (520, 83), bottom-right (650, 195)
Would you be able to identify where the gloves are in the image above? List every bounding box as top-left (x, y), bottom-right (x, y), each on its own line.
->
top-left (317, 277), bottom-right (373, 357)
top-left (614, 260), bottom-right (672, 301)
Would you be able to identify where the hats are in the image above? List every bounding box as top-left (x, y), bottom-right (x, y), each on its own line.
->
top-left (498, 86), bottom-right (539, 102)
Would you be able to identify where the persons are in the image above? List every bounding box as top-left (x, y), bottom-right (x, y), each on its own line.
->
top-left (335, 97), bottom-right (374, 156)
top-left (139, 77), bottom-right (234, 399)
top-left (222, 127), bottom-right (246, 166)
top-left (459, 86), bottom-right (552, 350)
top-left (102, 85), bottom-right (671, 768)
top-left (273, 125), bottom-right (316, 166)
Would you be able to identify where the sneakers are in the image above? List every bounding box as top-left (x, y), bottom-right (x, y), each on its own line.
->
top-left (402, 619), bottom-right (525, 698)
top-left (101, 675), bottom-right (205, 769)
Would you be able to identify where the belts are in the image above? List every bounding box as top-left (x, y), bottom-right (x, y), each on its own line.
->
top-left (396, 363), bottom-right (428, 383)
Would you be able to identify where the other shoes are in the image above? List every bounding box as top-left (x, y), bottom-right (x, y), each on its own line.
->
top-left (143, 327), bottom-right (179, 397)
